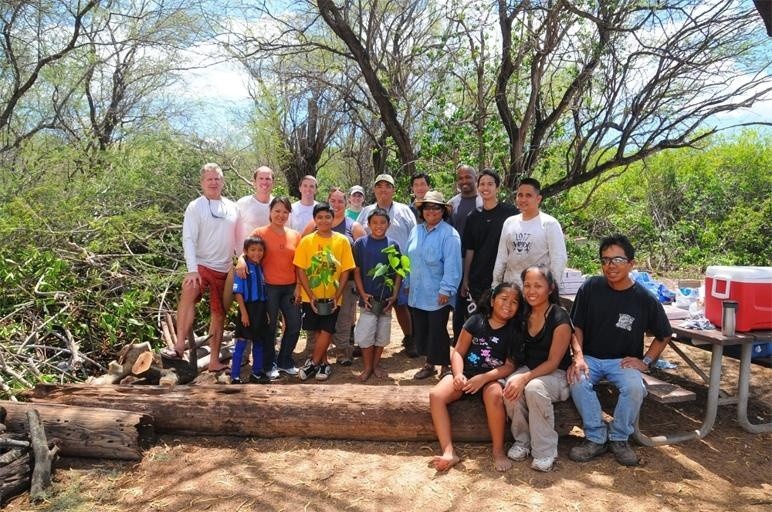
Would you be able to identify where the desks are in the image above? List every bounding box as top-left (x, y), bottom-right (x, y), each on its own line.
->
top-left (558, 291), bottom-right (772, 448)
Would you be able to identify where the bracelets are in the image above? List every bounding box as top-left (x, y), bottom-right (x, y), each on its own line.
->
top-left (641, 356), bottom-right (653, 365)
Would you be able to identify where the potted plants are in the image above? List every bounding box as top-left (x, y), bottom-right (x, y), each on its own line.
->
top-left (305, 244), bottom-right (342, 316)
top-left (367, 244), bottom-right (411, 314)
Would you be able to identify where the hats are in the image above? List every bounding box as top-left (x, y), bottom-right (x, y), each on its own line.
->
top-left (350, 185), bottom-right (364, 195)
top-left (374, 174), bottom-right (394, 187)
top-left (415, 190), bottom-right (449, 205)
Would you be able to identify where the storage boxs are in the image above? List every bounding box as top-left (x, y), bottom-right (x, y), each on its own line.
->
top-left (704, 266), bottom-right (772, 332)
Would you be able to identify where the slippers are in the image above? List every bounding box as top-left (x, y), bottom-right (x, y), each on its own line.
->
top-left (208, 365), bottom-right (230, 372)
top-left (161, 349), bottom-right (183, 361)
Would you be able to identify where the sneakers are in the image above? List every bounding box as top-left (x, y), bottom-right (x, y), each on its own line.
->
top-left (608, 440), bottom-right (637, 466)
top-left (403, 336), bottom-right (417, 357)
top-left (508, 442), bottom-right (530, 462)
top-left (231, 358), bottom-right (331, 384)
top-left (569, 439), bottom-right (608, 463)
top-left (530, 449), bottom-right (558, 473)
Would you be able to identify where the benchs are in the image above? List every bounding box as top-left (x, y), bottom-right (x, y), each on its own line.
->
top-left (669, 332), bottom-right (772, 372)
top-left (599, 373), bottom-right (696, 447)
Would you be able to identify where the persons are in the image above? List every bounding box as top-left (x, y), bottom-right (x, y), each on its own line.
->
top-left (424, 280), bottom-right (530, 473)
top-left (171, 161), bottom-right (568, 384)
top-left (566, 232), bottom-right (674, 469)
top-left (499, 262), bottom-right (577, 474)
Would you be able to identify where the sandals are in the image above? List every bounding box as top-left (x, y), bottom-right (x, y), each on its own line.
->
top-left (336, 353), bottom-right (352, 366)
top-left (414, 367), bottom-right (438, 380)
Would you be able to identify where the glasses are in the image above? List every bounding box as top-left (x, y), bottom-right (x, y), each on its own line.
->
top-left (208, 199), bottom-right (228, 219)
top-left (599, 257), bottom-right (629, 264)
top-left (422, 205), bottom-right (441, 210)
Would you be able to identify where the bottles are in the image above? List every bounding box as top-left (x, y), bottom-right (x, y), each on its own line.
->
top-left (721, 300), bottom-right (738, 337)
top-left (655, 360), bottom-right (678, 370)
